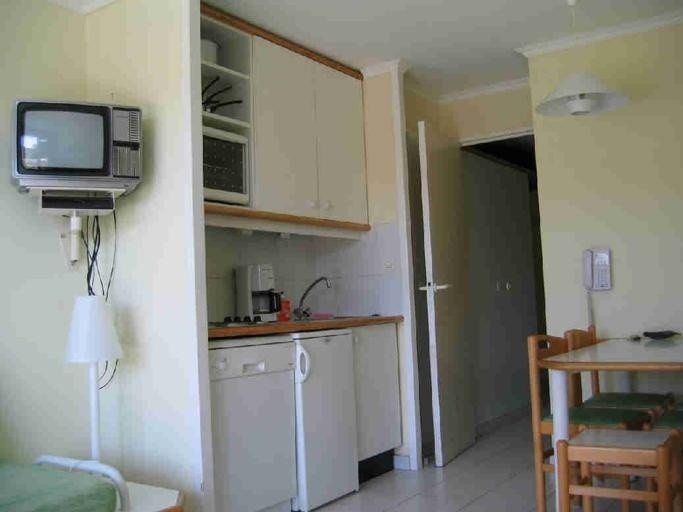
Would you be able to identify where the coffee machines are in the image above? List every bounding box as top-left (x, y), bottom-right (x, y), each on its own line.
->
top-left (232, 263), bottom-right (284, 323)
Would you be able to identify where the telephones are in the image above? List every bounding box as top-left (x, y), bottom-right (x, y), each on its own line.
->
top-left (583, 247), bottom-right (612, 327)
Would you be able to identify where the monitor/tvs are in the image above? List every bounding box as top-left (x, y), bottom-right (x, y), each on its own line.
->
top-left (11, 98), bottom-right (144, 193)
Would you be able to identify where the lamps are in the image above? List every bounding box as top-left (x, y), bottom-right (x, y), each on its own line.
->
top-left (200, 14), bottom-right (253, 216)
top-left (535, 1), bottom-right (624, 118)
top-left (65, 296), bottom-right (123, 460)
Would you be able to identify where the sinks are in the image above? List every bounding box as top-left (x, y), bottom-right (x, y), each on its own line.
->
top-left (288, 316), bottom-right (343, 322)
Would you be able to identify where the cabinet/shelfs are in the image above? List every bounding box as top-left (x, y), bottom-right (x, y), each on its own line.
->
top-left (253, 33), bottom-right (370, 228)
top-left (209, 338), bottom-right (299, 512)
top-left (294, 333), bottom-right (361, 512)
top-left (353, 322), bottom-right (402, 462)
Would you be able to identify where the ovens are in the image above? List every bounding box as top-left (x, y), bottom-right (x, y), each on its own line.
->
top-left (202, 125), bottom-right (250, 206)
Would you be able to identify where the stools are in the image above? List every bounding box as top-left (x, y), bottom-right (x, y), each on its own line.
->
top-left (556, 428), bottom-right (682, 511)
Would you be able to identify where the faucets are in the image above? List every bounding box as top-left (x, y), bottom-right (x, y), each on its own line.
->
top-left (293, 276), bottom-right (332, 318)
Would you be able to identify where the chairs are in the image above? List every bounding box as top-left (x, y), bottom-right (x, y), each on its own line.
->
top-left (527, 333), bottom-right (653, 507)
top-left (565, 325), bottom-right (671, 416)
